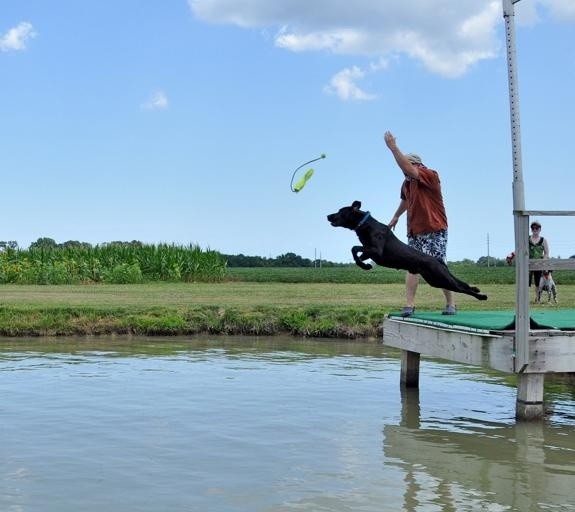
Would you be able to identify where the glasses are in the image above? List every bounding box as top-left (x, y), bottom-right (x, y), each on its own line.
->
top-left (531, 227), bottom-right (540, 230)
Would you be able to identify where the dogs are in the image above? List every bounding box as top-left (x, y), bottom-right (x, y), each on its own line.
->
top-left (326, 199), bottom-right (488, 302)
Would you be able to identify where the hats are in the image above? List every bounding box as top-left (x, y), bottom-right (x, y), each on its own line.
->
top-left (404, 152), bottom-right (428, 168)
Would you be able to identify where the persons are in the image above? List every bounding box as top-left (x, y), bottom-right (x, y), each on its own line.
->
top-left (384, 130), bottom-right (457, 315)
top-left (507, 222), bottom-right (549, 302)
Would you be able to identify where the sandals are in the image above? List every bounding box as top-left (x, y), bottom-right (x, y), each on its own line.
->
top-left (401, 304), bottom-right (456, 317)
